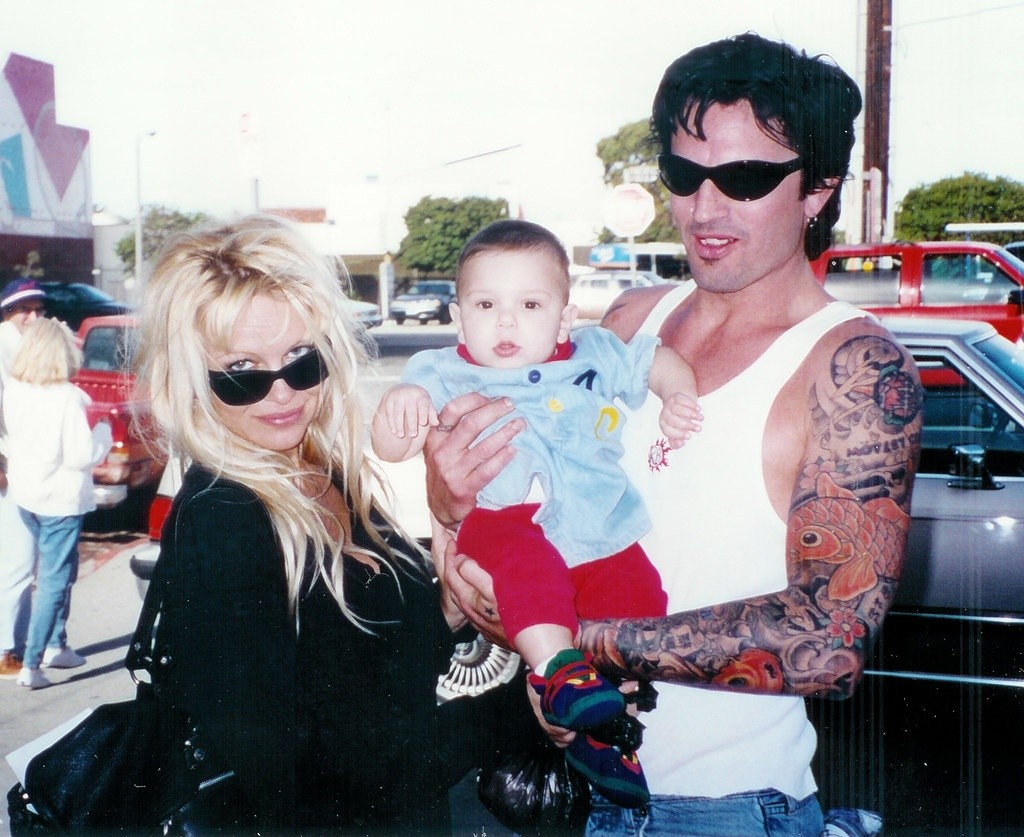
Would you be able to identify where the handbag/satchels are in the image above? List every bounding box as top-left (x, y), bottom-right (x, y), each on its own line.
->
top-left (476, 671), bottom-right (659, 837)
top-left (6, 479), bottom-right (315, 837)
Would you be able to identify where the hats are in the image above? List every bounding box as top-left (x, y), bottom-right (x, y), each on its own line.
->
top-left (0, 278), bottom-right (44, 307)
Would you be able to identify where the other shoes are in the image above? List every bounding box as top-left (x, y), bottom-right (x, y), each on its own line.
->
top-left (17, 668), bottom-right (51, 687)
top-left (529, 649), bottom-right (625, 729)
top-left (43, 645), bottom-right (87, 669)
top-left (564, 734), bottom-right (651, 808)
top-left (0, 652), bottom-right (23, 680)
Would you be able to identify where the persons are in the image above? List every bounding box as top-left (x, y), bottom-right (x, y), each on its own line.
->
top-left (0, 276), bottom-right (113, 688)
top-left (125, 215), bottom-right (470, 837)
top-left (368, 220), bottom-right (705, 809)
top-left (422, 34), bottom-right (923, 837)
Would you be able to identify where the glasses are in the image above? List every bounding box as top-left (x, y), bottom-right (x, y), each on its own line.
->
top-left (208, 347), bottom-right (331, 405)
top-left (656, 153), bottom-right (801, 201)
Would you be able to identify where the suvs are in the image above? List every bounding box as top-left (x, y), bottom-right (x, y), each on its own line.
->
top-left (806, 240), bottom-right (1024, 391)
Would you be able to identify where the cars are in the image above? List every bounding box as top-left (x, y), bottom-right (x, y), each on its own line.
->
top-left (566, 240), bottom-right (695, 327)
top-left (0, 280), bottom-right (201, 600)
top-left (865, 314), bottom-right (1024, 675)
top-left (946, 222), bottom-right (1024, 270)
top-left (347, 297), bottom-right (384, 330)
top-left (392, 279), bottom-right (459, 327)
